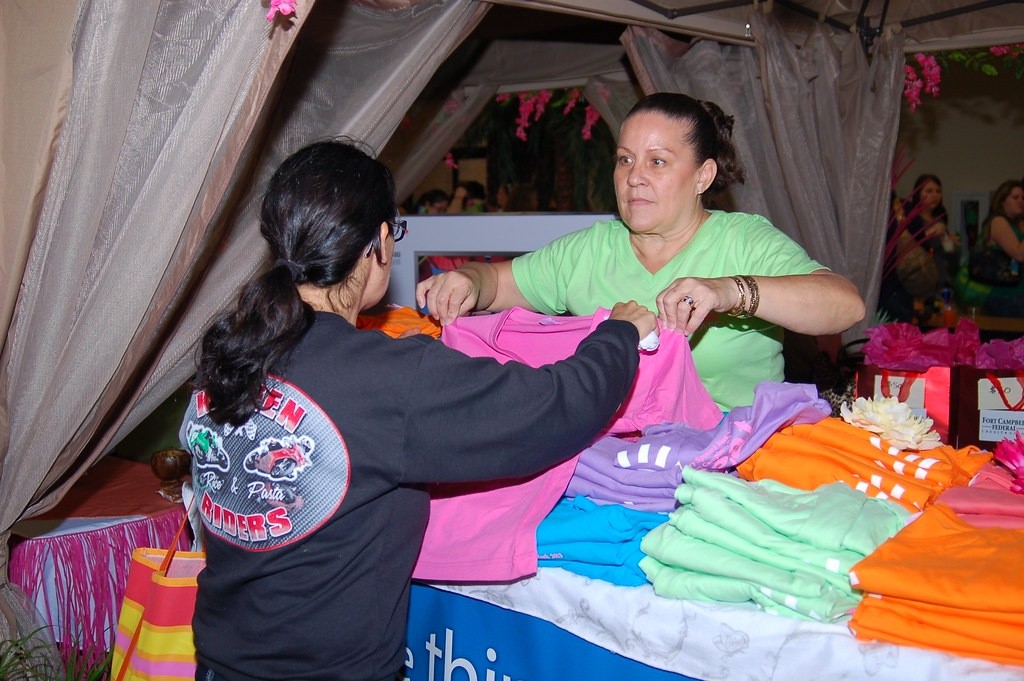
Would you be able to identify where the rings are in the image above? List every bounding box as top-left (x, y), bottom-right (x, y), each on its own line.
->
top-left (681, 295), bottom-right (696, 310)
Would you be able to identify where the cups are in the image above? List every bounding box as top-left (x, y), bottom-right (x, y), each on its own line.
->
top-left (940, 288), bottom-right (953, 303)
top-left (968, 307), bottom-right (980, 323)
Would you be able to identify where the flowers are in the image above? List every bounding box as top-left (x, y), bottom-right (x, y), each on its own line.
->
top-left (267, 0), bottom-right (298, 22)
top-left (400, 86), bottom-right (613, 213)
top-left (904, 45), bottom-right (1024, 115)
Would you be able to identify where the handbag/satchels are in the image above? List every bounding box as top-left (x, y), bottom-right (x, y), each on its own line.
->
top-left (855, 362), bottom-right (957, 447)
top-left (109, 494), bottom-right (207, 681)
top-left (955, 367), bottom-right (1024, 451)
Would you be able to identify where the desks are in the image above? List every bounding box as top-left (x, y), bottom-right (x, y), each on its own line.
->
top-left (11, 455), bottom-right (193, 677)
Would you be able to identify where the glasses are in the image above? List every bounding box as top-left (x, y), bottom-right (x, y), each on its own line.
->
top-left (364, 219), bottom-right (407, 258)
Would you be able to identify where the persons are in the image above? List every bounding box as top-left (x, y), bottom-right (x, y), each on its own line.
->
top-left (883, 173), bottom-right (961, 334)
top-left (177, 134), bottom-right (658, 681)
top-left (415, 91), bottom-right (867, 414)
top-left (963, 178), bottom-right (1024, 344)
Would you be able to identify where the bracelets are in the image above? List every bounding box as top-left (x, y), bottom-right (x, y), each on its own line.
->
top-left (723, 274), bottom-right (760, 320)
